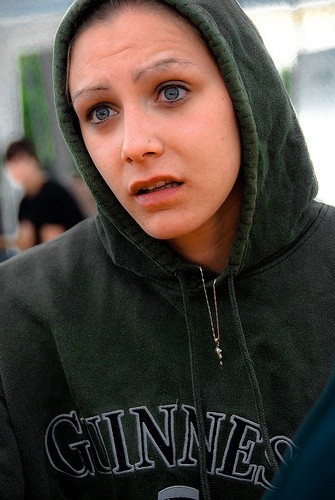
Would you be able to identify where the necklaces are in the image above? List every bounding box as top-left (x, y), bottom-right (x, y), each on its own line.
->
top-left (197, 264), bottom-right (224, 367)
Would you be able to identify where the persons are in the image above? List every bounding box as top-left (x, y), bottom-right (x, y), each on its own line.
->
top-left (0, 139), bottom-right (84, 254)
top-left (1, 1), bottom-right (335, 500)
